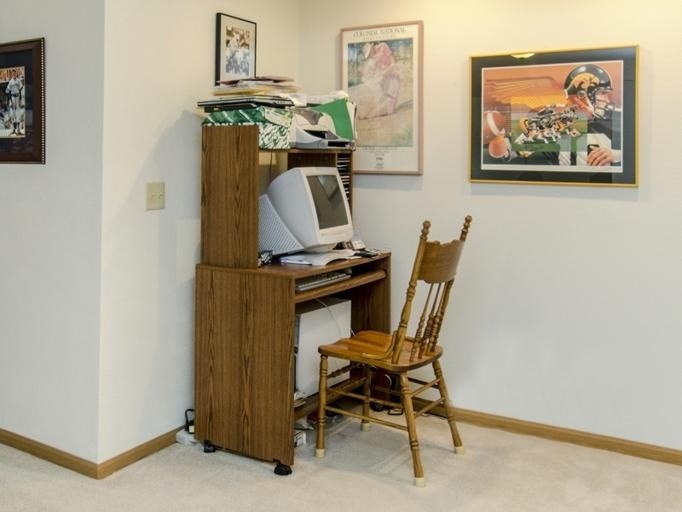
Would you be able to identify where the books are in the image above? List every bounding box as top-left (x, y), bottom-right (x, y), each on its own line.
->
top-left (195, 75), bottom-right (293, 106)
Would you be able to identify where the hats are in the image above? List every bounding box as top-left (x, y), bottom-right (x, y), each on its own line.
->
top-left (362, 43), bottom-right (374, 57)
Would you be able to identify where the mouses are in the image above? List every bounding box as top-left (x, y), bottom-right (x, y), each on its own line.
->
top-left (344, 268), bottom-right (353, 274)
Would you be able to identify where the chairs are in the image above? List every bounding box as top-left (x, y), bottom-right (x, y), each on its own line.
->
top-left (314, 214), bottom-right (471, 488)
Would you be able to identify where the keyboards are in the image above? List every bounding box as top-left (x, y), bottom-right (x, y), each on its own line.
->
top-left (295, 272), bottom-right (352, 294)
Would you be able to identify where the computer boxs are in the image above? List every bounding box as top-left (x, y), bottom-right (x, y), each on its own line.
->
top-left (294, 295), bottom-right (352, 399)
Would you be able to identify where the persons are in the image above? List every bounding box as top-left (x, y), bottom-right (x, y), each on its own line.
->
top-left (488, 63), bottom-right (621, 167)
top-left (359, 40), bottom-right (401, 119)
top-left (0, 69), bottom-right (26, 135)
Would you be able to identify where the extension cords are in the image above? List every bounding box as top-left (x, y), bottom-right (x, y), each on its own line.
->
top-left (176, 429), bottom-right (197, 447)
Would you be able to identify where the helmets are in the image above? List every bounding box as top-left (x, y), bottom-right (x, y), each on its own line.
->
top-left (563, 62), bottom-right (615, 121)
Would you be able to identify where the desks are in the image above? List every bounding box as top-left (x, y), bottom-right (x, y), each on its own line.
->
top-left (195, 254), bottom-right (393, 461)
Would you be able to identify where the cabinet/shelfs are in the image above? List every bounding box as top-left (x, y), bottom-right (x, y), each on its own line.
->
top-left (200, 124), bottom-right (354, 261)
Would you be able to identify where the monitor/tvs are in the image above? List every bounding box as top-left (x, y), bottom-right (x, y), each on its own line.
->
top-left (258, 166), bottom-right (359, 266)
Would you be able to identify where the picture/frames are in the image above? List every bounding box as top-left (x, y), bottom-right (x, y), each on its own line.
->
top-left (466, 44), bottom-right (642, 190)
top-left (339, 19), bottom-right (423, 177)
top-left (215, 12), bottom-right (257, 86)
top-left (0, 37), bottom-right (47, 166)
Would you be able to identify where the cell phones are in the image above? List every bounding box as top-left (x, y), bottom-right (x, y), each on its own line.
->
top-left (356, 248), bottom-right (379, 258)
